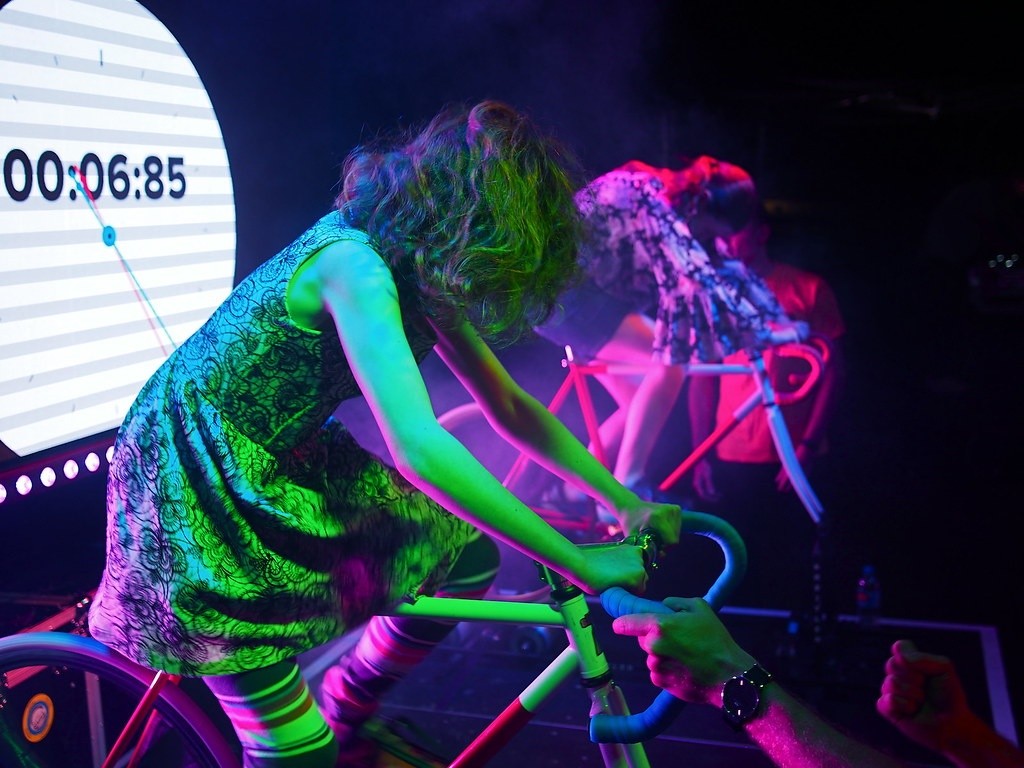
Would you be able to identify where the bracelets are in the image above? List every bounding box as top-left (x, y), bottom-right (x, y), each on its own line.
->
top-left (800, 439), bottom-right (819, 450)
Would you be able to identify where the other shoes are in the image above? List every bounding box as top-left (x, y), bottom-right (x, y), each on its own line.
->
top-left (541, 487), bottom-right (590, 522)
top-left (596, 473), bottom-right (680, 536)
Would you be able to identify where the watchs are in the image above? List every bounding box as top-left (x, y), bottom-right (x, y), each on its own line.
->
top-left (721, 663), bottom-right (774, 727)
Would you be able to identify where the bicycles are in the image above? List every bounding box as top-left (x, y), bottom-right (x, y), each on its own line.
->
top-left (0, 507), bottom-right (745, 768)
top-left (434, 331), bottom-right (830, 601)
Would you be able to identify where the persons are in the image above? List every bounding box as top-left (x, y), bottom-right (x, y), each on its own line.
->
top-left (612, 597), bottom-right (1024, 768)
top-left (687, 207), bottom-right (847, 720)
top-left (533, 155), bottom-right (809, 526)
top-left (89, 99), bottom-right (682, 768)
